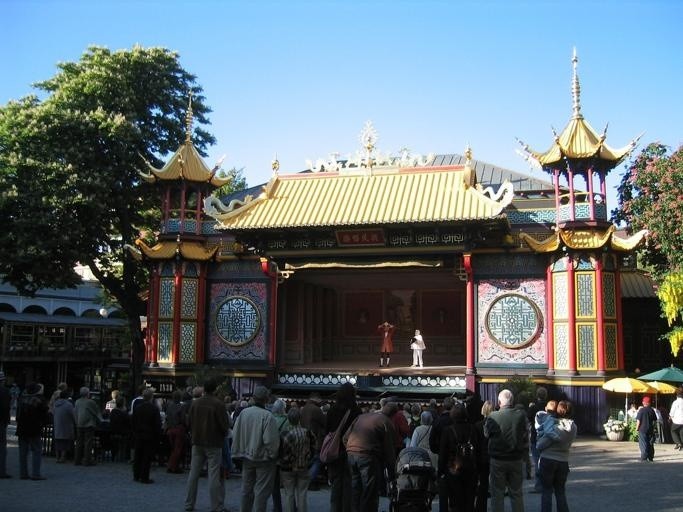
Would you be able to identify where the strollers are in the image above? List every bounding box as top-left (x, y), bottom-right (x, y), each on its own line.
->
top-left (385, 447), bottom-right (437, 511)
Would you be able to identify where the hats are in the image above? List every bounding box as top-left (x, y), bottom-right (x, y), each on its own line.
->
top-left (643, 397), bottom-right (650, 403)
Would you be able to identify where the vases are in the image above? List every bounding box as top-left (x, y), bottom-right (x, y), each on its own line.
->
top-left (603, 431), bottom-right (625, 441)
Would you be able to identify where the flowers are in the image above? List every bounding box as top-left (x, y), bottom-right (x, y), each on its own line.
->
top-left (602, 419), bottom-right (629, 433)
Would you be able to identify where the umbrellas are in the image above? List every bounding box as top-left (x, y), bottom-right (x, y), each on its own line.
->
top-left (603, 364), bottom-right (683, 425)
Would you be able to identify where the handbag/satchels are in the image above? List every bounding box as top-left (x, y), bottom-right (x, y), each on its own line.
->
top-left (320, 432), bottom-right (340, 463)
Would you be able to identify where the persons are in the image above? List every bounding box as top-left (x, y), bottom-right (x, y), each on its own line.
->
top-left (668, 392), bottom-right (683, 451)
top-left (409, 329), bottom-right (427, 367)
top-left (378, 321), bottom-right (397, 367)
top-left (184, 379), bottom-right (576, 512)
top-left (635, 396), bottom-right (657, 462)
top-left (0, 376), bottom-right (204, 485)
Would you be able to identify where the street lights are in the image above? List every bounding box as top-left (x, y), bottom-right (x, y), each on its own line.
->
top-left (97, 298), bottom-right (108, 410)
